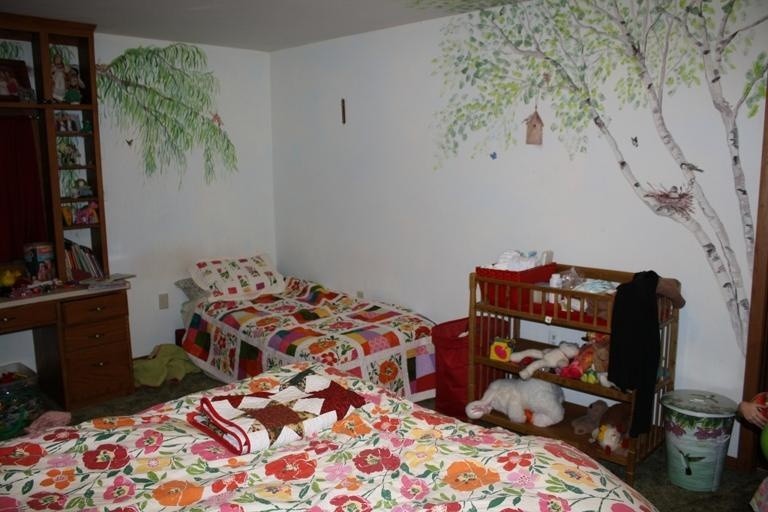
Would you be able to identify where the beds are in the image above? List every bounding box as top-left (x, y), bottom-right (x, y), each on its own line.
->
top-left (1, 364), bottom-right (667, 511)
top-left (175, 275), bottom-right (451, 399)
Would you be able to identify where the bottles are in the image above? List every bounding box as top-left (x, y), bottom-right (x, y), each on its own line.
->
top-left (548, 274), bottom-right (563, 302)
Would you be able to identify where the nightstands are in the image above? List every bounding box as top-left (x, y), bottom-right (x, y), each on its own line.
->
top-left (431, 314), bottom-right (513, 421)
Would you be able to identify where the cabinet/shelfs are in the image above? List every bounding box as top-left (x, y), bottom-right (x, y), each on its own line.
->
top-left (467, 263), bottom-right (682, 486)
top-left (0, 10), bottom-right (139, 415)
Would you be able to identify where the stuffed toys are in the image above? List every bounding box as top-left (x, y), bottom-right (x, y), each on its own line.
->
top-left (483, 330), bottom-right (614, 389)
top-left (591, 402), bottom-right (635, 454)
top-left (572, 399), bottom-right (608, 437)
top-left (464, 376), bottom-right (568, 429)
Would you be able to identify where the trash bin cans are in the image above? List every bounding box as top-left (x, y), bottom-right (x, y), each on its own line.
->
top-left (660, 389), bottom-right (740, 492)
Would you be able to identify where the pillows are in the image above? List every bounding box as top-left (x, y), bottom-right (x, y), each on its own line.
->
top-left (189, 256), bottom-right (294, 300)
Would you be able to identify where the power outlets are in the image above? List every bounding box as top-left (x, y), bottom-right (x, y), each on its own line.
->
top-left (158, 293), bottom-right (169, 310)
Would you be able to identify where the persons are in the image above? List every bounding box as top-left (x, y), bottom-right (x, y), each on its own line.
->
top-left (64, 66), bottom-right (86, 105)
top-left (0, 71), bottom-right (11, 95)
top-left (51, 53), bottom-right (68, 103)
top-left (6, 72), bottom-right (26, 97)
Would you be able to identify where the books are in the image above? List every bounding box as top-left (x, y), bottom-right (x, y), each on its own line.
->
top-left (19, 239), bottom-right (105, 281)
top-left (79, 272), bottom-right (136, 286)
